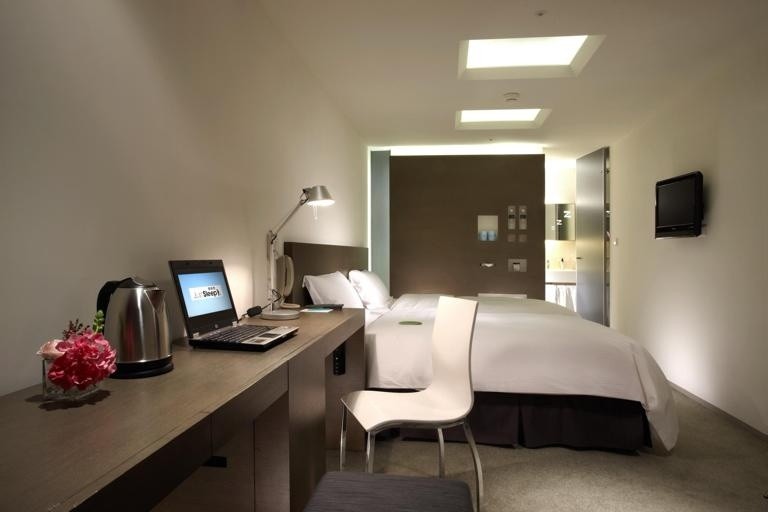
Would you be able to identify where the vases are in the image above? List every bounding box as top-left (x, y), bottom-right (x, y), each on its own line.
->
top-left (39, 357), bottom-right (104, 400)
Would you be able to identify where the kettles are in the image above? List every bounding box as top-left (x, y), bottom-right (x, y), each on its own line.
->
top-left (96, 277), bottom-right (175, 380)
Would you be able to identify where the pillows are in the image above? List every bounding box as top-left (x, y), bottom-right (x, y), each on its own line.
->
top-left (301, 270), bottom-right (365, 308)
top-left (348, 269), bottom-right (394, 307)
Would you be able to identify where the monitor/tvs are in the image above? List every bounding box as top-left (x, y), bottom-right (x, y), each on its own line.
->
top-left (655, 171), bottom-right (705, 238)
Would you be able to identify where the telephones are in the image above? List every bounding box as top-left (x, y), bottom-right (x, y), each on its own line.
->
top-left (276, 256), bottom-right (295, 301)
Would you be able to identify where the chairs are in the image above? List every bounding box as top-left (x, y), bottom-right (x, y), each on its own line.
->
top-left (339, 295), bottom-right (487, 510)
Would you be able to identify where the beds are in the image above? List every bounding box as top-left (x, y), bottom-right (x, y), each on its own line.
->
top-left (282, 240), bottom-right (680, 457)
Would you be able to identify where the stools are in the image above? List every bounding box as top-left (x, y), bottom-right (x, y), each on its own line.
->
top-left (300, 469), bottom-right (475, 512)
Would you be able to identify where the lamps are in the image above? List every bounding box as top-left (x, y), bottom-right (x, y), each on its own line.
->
top-left (260, 183), bottom-right (336, 321)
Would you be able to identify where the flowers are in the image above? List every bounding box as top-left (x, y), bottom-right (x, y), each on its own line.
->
top-left (37, 311), bottom-right (118, 391)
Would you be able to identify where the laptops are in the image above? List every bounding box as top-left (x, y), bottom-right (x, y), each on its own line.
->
top-left (168, 259), bottom-right (300, 352)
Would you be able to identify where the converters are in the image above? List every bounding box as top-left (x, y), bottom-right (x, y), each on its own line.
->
top-left (248, 306), bottom-right (262, 318)
